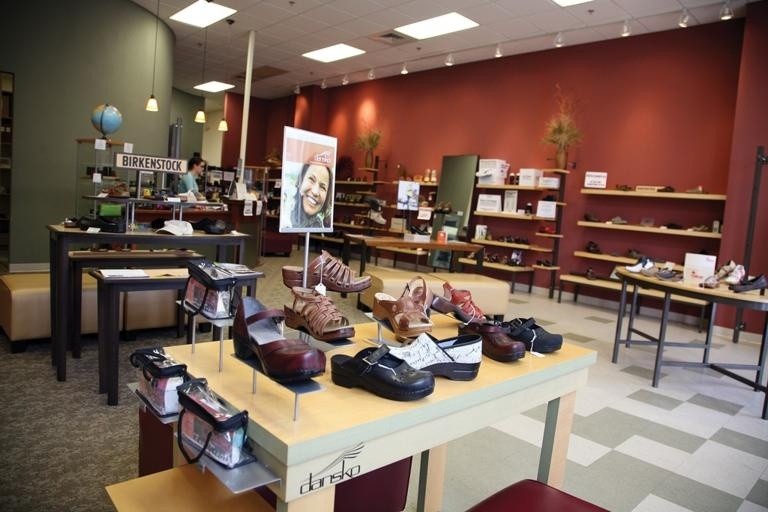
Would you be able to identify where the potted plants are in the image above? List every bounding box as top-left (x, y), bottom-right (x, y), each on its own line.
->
top-left (351, 127), bottom-right (381, 168)
top-left (537, 83), bottom-right (582, 170)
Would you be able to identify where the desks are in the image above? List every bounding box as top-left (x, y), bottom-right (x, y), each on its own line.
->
top-left (609, 265), bottom-right (767, 420)
top-left (161, 319), bottom-right (598, 512)
top-left (341, 233), bottom-right (485, 309)
top-left (46, 223), bottom-right (253, 384)
top-left (66, 248), bottom-right (208, 361)
top-left (88, 268), bottom-right (266, 407)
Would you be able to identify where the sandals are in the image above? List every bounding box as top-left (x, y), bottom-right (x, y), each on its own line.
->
top-left (282, 249), bottom-right (372, 341)
top-left (435, 283), bottom-right (484, 320)
top-left (374, 275), bottom-right (434, 337)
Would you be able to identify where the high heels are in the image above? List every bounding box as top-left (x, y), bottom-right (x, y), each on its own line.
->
top-left (410, 225), bottom-right (430, 235)
top-left (233, 298), bottom-right (326, 382)
top-left (627, 256), bottom-right (767, 294)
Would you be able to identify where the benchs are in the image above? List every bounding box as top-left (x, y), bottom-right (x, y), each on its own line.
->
top-left (297, 233), bottom-right (363, 251)
top-left (556, 273), bottom-right (710, 333)
top-left (374, 245), bottom-right (428, 271)
top-left (458, 257), bottom-right (534, 294)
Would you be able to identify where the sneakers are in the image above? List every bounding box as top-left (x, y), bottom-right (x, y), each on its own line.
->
top-left (615, 185), bottom-right (705, 193)
top-left (64, 181), bottom-right (231, 235)
top-left (584, 212), bottom-right (710, 231)
top-left (539, 225), bottom-right (555, 233)
top-left (543, 195), bottom-right (555, 201)
top-left (536, 258), bottom-right (551, 265)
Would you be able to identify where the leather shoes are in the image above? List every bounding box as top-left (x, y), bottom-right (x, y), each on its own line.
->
top-left (330, 333), bottom-right (483, 400)
top-left (463, 318), bottom-right (563, 362)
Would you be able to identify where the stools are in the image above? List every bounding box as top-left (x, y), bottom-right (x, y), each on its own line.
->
top-left (127, 290), bottom-right (211, 341)
top-left (465, 477), bottom-right (610, 512)
top-left (358, 271), bottom-right (448, 315)
top-left (262, 233), bottom-right (292, 257)
top-left (428, 272), bottom-right (510, 323)
top-left (2, 272), bottom-right (126, 352)
top-left (137, 392), bottom-right (412, 512)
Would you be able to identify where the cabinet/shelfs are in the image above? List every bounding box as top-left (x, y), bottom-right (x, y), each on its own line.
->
top-left (75, 137), bottom-right (130, 224)
top-left (334, 179), bottom-right (438, 235)
top-left (265, 166), bottom-right (282, 220)
top-left (467, 168), bottom-right (569, 271)
top-left (572, 186), bottom-right (726, 274)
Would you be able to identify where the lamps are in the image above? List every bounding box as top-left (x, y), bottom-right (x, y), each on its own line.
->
top-left (217, 89), bottom-right (230, 131)
top-left (290, 0), bottom-right (734, 97)
top-left (145, 0), bottom-right (159, 112)
top-left (193, 27), bottom-right (208, 123)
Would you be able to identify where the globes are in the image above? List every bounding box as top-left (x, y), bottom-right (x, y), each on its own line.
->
top-left (91, 103), bottom-right (123, 140)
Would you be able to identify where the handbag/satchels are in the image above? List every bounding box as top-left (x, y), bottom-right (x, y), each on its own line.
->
top-left (128, 348), bottom-right (187, 418)
top-left (509, 249), bottom-right (524, 266)
top-left (180, 260), bottom-right (237, 321)
top-left (175, 379), bottom-right (256, 470)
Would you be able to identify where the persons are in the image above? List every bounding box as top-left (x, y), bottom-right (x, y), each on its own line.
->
top-left (177, 151), bottom-right (212, 230)
top-left (287, 157), bottom-right (332, 228)
top-left (402, 188), bottom-right (416, 210)
